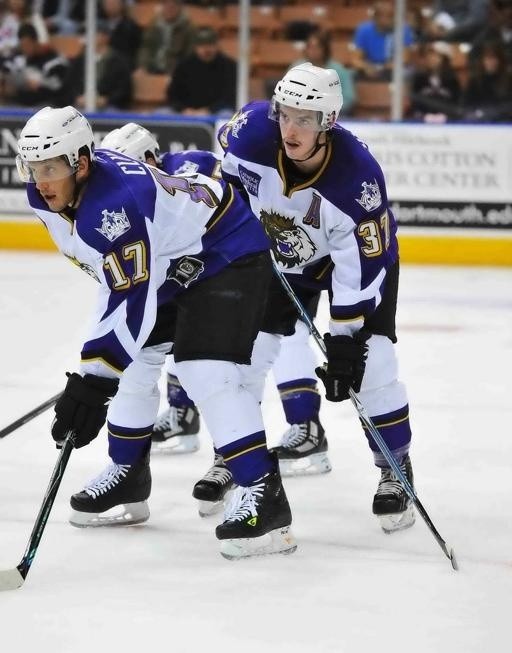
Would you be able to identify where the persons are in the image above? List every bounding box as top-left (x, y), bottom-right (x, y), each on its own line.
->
top-left (98, 122), bottom-right (332, 460)
top-left (1, 0), bottom-right (511, 129)
top-left (10, 103), bottom-right (299, 539)
top-left (194, 57), bottom-right (416, 516)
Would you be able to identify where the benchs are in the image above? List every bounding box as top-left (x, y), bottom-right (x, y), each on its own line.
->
top-left (0, 0), bottom-right (511, 128)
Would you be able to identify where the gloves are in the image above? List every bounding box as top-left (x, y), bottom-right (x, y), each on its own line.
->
top-left (315, 329), bottom-right (371, 403)
top-left (48, 371), bottom-right (119, 449)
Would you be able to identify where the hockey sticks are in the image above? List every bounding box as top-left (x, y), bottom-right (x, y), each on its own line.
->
top-left (1, 430), bottom-right (77, 590)
top-left (270, 247), bottom-right (459, 571)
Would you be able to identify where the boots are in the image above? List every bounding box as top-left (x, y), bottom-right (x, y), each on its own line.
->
top-left (371, 452), bottom-right (415, 514)
top-left (149, 399), bottom-right (202, 442)
top-left (190, 444), bottom-right (236, 501)
top-left (68, 432), bottom-right (154, 513)
top-left (269, 394), bottom-right (330, 460)
top-left (212, 451), bottom-right (295, 540)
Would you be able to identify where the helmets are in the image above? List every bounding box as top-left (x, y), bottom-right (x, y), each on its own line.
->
top-left (100, 120), bottom-right (159, 166)
top-left (13, 103), bottom-right (95, 184)
top-left (266, 60), bottom-right (345, 134)
